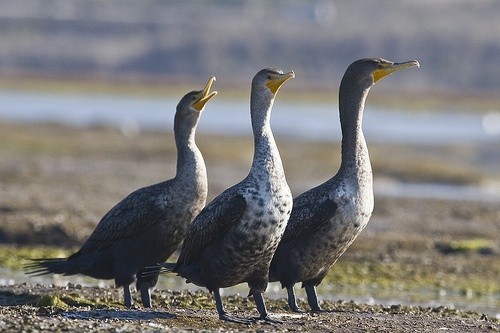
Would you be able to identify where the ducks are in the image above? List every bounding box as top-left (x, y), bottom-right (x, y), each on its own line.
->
top-left (246, 56), bottom-right (421, 317)
top-left (143, 67), bottom-right (296, 326)
top-left (24, 72), bottom-right (218, 312)
